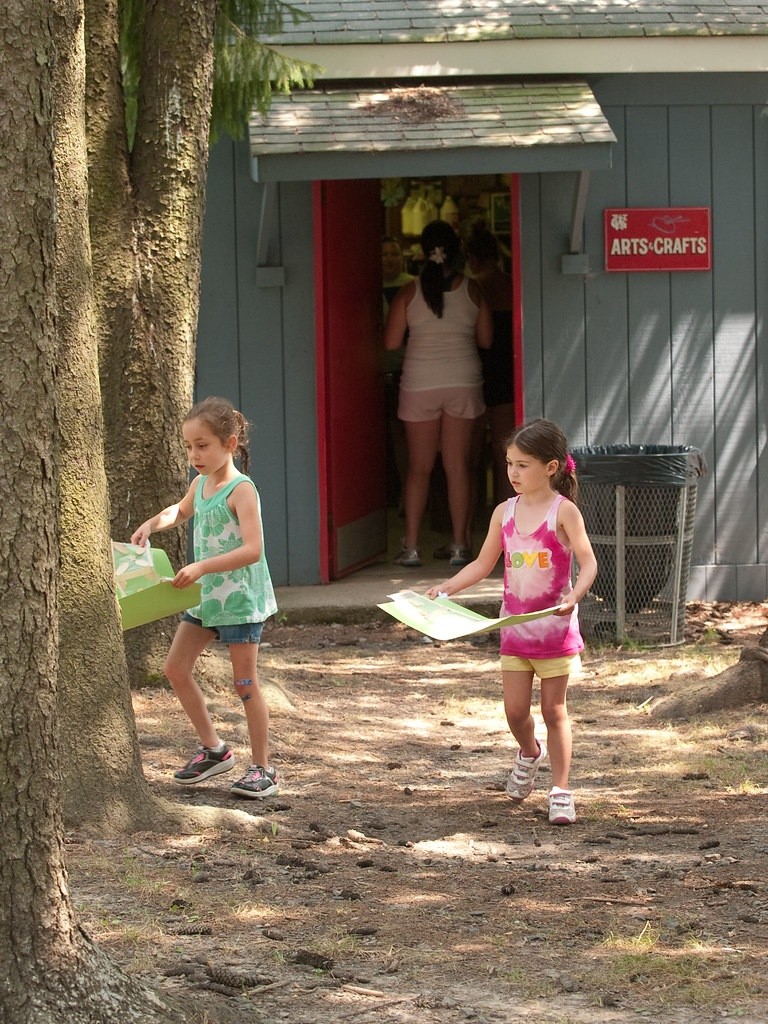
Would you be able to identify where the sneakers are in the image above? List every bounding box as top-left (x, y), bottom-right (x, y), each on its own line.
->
top-left (231, 764), bottom-right (279, 798)
top-left (548, 786), bottom-right (576, 825)
top-left (505, 737), bottom-right (546, 802)
top-left (393, 543), bottom-right (422, 566)
top-left (174, 741), bottom-right (235, 784)
top-left (448, 543), bottom-right (473, 565)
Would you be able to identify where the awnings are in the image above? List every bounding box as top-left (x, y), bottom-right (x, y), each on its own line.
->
top-left (246, 83), bottom-right (617, 263)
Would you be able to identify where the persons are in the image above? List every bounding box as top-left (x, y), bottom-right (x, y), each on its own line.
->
top-left (383, 220), bottom-right (494, 567)
top-left (424, 418), bottom-right (598, 823)
top-left (131, 395), bottom-right (279, 799)
top-left (378, 219), bottom-right (514, 559)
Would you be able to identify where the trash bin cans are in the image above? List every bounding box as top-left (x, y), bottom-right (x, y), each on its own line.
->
top-left (570, 446), bottom-right (706, 647)
